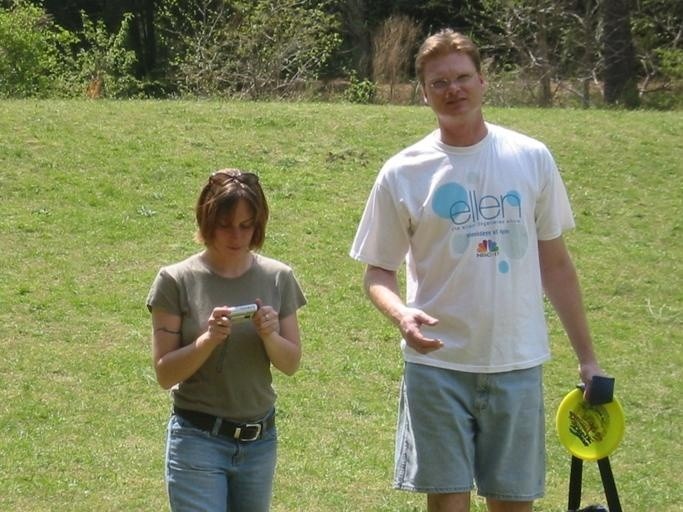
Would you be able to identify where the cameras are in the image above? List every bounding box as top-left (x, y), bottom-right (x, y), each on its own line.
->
top-left (224, 303), bottom-right (259, 322)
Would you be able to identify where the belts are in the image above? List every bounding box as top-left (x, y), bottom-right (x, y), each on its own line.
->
top-left (172, 404), bottom-right (275, 443)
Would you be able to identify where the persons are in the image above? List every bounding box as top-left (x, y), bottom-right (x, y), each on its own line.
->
top-left (144, 166), bottom-right (307, 512)
top-left (349, 27), bottom-right (616, 512)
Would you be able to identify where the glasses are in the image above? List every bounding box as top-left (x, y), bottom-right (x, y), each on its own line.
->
top-left (423, 70), bottom-right (477, 89)
top-left (208, 172), bottom-right (260, 193)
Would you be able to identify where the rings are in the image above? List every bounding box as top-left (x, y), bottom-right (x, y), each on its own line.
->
top-left (263, 314), bottom-right (269, 322)
top-left (215, 317), bottom-right (223, 326)
